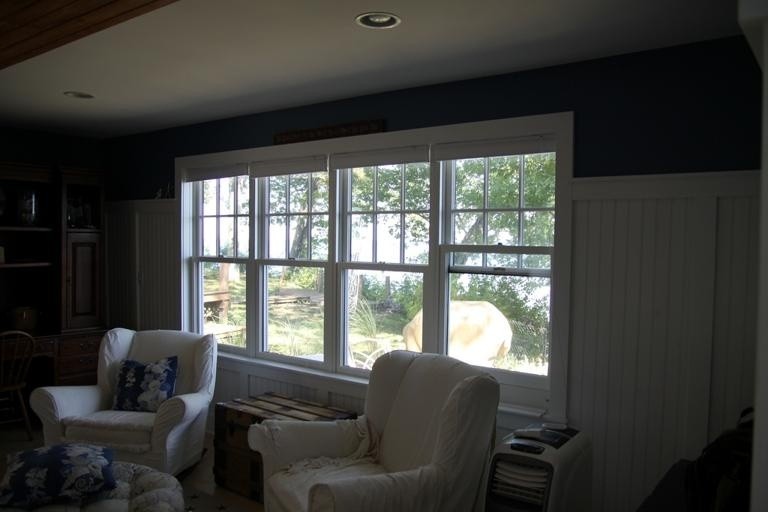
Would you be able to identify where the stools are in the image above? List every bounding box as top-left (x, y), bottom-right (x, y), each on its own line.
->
top-left (0, 459), bottom-right (186, 512)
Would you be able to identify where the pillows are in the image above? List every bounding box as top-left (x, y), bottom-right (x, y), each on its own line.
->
top-left (0, 440), bottom-right (118, 510)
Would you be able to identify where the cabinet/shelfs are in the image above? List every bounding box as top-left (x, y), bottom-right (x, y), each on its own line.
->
top-left (1, 165), bottom-right (113, 383)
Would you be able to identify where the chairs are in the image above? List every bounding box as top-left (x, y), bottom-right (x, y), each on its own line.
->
top-left (0, 325), bottom-right (217, 477)
top-left (245, 351), bottom-right (499, 510)
